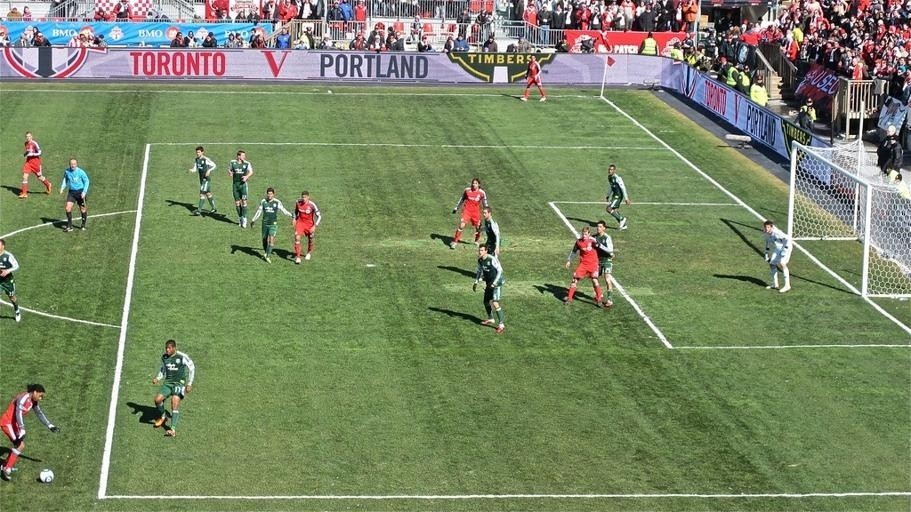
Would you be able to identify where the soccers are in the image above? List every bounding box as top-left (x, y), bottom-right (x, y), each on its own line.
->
top-left (40, 469), bottom-right (53, 483)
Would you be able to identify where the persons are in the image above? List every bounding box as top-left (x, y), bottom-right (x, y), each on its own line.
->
top-left (592, 220), bottom-right (616, 307)
top-left (186, 146), bottom-right (321, 265)
top-left (59, 157), bottom-right (90, 232)
top-left (520, 55), bottom-right (546, 102)
top-left (449, 176), bottom-right (505, 335)
top-left (876, 125), bottom-right (904, 181)
top-left (2, 1), bottom-right (697, 55)
top-left (151, 339), bottom-right (196, 437)
top-left (605, 164), bottom-right (631, 233)
top-left (18, 130), bottom-right (52, 199)
top-left (0, 239), bottom-right (22, 323)
top-left (0, 382), bottom-right (62, 480)
top-left (563, 227), bottom-right (605, 308)
top-left (761, 220), bottom-right (795, 293)
top-left (668, 0), bottom-right (910, 134)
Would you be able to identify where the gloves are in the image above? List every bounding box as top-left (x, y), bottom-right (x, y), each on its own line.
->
top-left (19, 429), bottom-right (25, 441)
top-left (49, 424), bottom-right (60, 433)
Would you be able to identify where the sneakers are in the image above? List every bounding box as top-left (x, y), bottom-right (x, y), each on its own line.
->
top-left (46, 184), bottom-right (51, 194)
top-left (521, 96), bottom-right (546, 102)
top-left (474, 242), bottom-right (479, 247)
top-left (15, 309), bottom-right (22, 323)
top-left (63, 227), bottom-right (72, 232)
top-left (154, 415), bottom-right (167, 427)
top-left (1, 464), bottom-right (19, 481)
top-left (450, 241), bottom-right (457, 249)
top-left (482, 319), bottom-right (504, 332)
top-left (18, 192), bottom-right (28, 198)
top-left (595, 295), bottom-right (612, 307)
top-left (765, 283), bottom-right (793, 294)
top-left (617, 217), bottom-right (628, 232)
top-left (264, 253), bottom-right (310, 264)
top-left (81, 224), bottom-right (85, 230)
top-left (193, 209), bottom-right (247, 230)
top-left (166, 430), bottom-right (176, 437)
